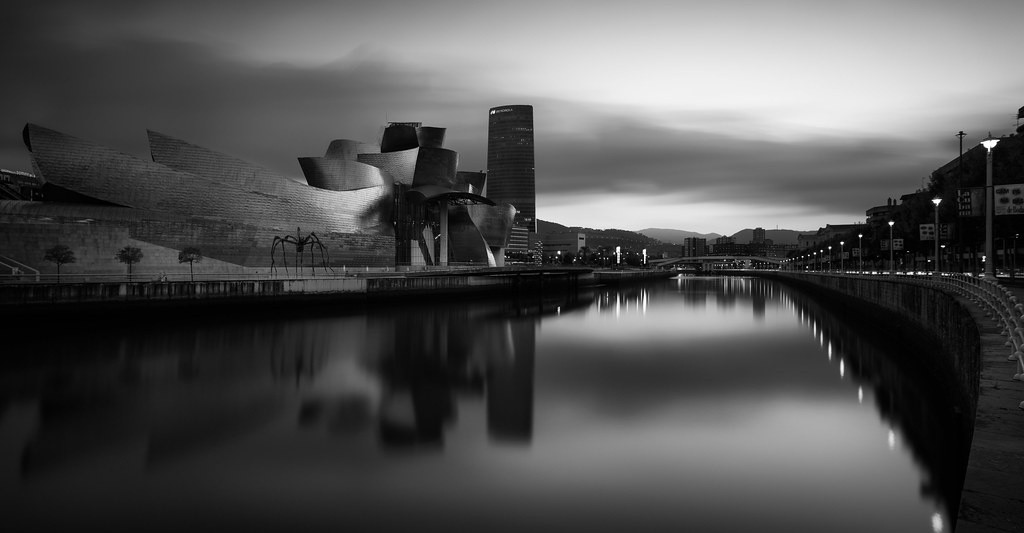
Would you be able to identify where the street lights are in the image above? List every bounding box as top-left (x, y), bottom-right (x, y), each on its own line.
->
top-left (887, 219), bottom-right (896, 275)
top-left (779, 246), bottom-right (832, 271)
top-left (838, 241), bottom-right (846, 272)
top-left (858, 233), bottom-right (863, 275)
top-left (931, 195), bottom-right (943, 275)
top-left (955, 130), bottom-right (968, 188)
top-left (980, 131), bottom-right (1001, 282)
